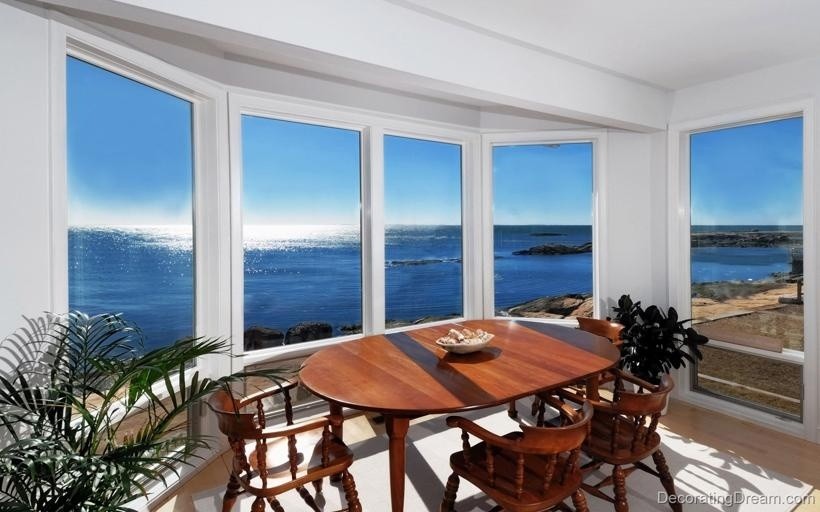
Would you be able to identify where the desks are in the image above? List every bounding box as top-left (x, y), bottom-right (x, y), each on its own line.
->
top-left (299, 319), bottom-right (620, 512)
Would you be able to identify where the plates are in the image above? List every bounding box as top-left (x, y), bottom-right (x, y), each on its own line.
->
top-left (436, 334), bottom-right (496, 354)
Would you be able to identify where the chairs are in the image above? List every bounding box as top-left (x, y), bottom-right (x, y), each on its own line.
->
top-left (208, 379), bottom-right (366, 512)
top-left (436, 312), bottom-right (686, 511)
